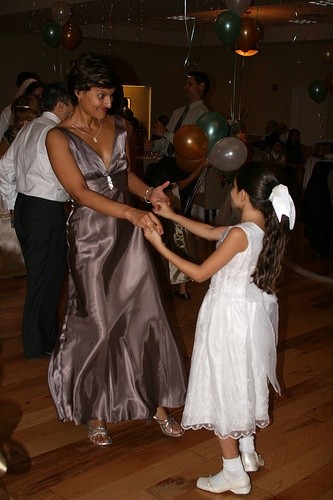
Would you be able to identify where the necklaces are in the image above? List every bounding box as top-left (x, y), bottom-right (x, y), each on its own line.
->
top-left (69, 111), bottom-right (102, 144)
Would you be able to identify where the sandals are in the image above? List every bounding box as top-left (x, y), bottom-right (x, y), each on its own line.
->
top-left (87, 420), bottom-right (112, 445)
top-left (151, 411), bottom-right (185, 437)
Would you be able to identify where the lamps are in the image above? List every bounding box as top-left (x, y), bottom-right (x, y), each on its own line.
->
top-left (234, 18), bottom-right (264, 57)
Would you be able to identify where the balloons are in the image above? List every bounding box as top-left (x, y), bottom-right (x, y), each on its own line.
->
top-left (308, 79), bottom-right (327, 104)
top-left (173, 112), bottom-right (248, 173)
top-left (214, 0), bottom-right (264, 58)
top-left (44, 2), bottom-right (82, 50)
top-left (326, 72), bottom-right (333, 95)
top-left (320, 44), bottom-right (333, 65)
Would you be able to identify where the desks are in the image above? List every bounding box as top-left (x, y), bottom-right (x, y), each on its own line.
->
top-left (300, 155), bottom-right (333, 259)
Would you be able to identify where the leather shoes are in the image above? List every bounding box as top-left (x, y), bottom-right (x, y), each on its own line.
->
top-left (196, 471), bottom-right (251, 494)
top-left (38, 352), bottom-right (52, 357)
top-left (175, 290), bottom-right (190, 300)
top-left (240, 451), bottom-right (264, 471)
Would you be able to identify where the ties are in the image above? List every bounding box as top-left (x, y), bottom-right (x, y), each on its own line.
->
top-left (173, 105), bottom-right (190, 133)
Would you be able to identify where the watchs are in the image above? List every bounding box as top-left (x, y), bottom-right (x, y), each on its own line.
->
top-left (163, 130), bottom-right (170, 138)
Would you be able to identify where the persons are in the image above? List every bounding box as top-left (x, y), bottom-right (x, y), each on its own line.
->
top-left (146, 156), bottom-right (208, 300)
top-left (46, 56), bottom-right (185, 446)
top-left (144, 168), bottom-right (295, 495)
top-left (144, 72), bottom-right (210, 182)
top-left (250, 120), bottom-right (306, 163)
top-left (152, 116), bottom-right (168, 141)
top-left (0, 71), bottom-right (78, 357)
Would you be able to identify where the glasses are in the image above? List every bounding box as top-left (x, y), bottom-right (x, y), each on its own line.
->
top-left (34, 95), bottom-right (42, 100)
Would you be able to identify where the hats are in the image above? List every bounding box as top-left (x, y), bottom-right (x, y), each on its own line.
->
top-left (144, 156), bottom-right (178, 188)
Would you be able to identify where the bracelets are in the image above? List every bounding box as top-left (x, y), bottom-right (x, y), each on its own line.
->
top-left (144, 187), bottom-right (154, 204)
